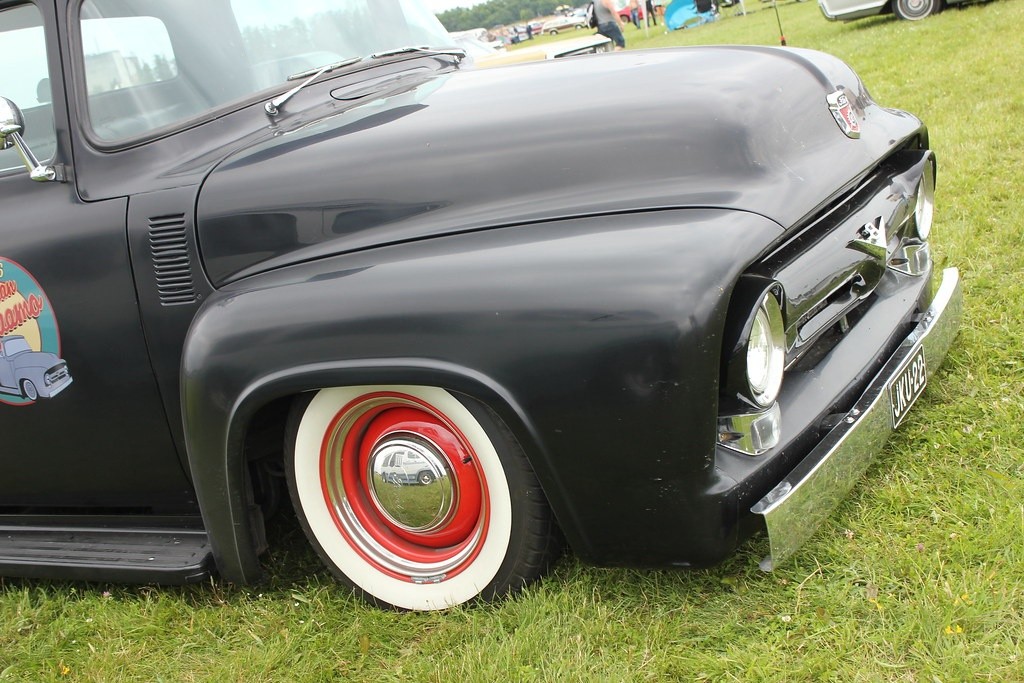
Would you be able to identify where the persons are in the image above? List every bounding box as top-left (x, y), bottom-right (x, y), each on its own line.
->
top-left (511, 25), bottom-right (521, 42)
top-left (594, 0), bottom-right (626, 53)
top-left (525, 21), bottom-right (535, 40)
top-left (629, 0), bottom-right (643, 30)
top-left (653, 1), bottom-right (665, 26)
top-left (645, 0), bottom-right (657, 28)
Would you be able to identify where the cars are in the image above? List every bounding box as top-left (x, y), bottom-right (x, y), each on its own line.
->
top-left (526, 21), bottom-right (544, 35)
top-left (1, 1), bottom-right (962, 623)
top-left (541, 17), bottom-right (587, 35)
top-left (615, 4), bottom-right (645, 23)
top-left (818, 0), bottom-right (984, 22)
top-left (507, 24), bottom-right (529, 38)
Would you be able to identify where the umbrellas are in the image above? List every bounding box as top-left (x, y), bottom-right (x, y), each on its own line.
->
top-left (556, 3), bottom-right (571, 12)
